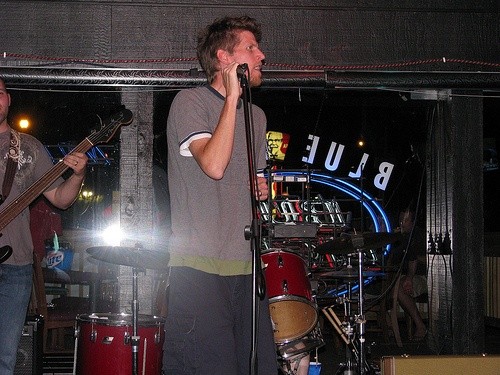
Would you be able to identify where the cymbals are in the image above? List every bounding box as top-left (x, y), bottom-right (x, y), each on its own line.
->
top-left (313, 231), bottom-right (401, 254)
top-left (86, 246), bottom-right (170, 269)
top-left (321, 267), bottom-right (389, 278)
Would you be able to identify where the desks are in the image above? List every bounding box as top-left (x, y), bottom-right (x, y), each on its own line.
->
top-left (41, 268), bottom-right (115, 315)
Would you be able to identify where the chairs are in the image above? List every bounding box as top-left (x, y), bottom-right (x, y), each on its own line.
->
top-left (32, 267), bottom-right (79, 353)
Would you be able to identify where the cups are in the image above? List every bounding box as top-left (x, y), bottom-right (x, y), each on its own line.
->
top-left (308, 362), bottom-right (321, 375)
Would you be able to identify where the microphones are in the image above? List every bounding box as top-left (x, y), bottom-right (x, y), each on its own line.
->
top-left (236, 63), bottom-right (248, 78)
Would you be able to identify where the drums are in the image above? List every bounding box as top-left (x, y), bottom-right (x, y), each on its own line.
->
top-left (259, 247), bottom-right (318, 344)
top-left (77, 312), bottom-right (166, 375)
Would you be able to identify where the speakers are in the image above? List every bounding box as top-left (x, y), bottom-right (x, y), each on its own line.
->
top-left (13, 315), bottom-right (44, 375)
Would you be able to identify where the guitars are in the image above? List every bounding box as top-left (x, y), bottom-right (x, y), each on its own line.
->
top-left (0, 102), bottom-right (135, 263)
